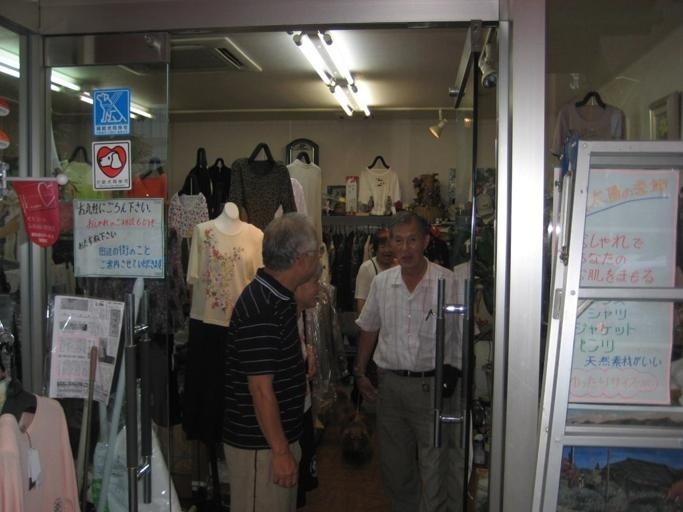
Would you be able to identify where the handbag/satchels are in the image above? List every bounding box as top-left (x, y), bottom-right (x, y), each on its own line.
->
top-left (299, 440), bottom-right (319, 491)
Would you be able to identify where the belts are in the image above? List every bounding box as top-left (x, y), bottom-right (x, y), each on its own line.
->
top-left (390, 369), bottom-right (436, 377)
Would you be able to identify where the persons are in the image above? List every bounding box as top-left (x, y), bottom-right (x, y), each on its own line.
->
top-left (221, 213), bottom-right (319, 511)
top-left (353, 213), bottom-right (479, 510)
top-left (293, 261), bottom-right (323, 508)
top-left (355, 230), bottom-right (400, 316)
top-left (179, 201), bottom-right (264, 446)
top-left (424, 233), bottom-right (450, 269)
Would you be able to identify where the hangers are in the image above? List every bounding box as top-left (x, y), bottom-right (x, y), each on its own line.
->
top-left (1, 340), bottom-right (40, 419)
top-left (367, 156), bottom-right (391, 169)
top-left (321, 223), bottom-right (386, 244)
top-left (248, 142), bottom-right (274, 164)
top-left (195, 158), bottom-right (226, 170)
top-left (69, 144), bottom-right (89, 162)
top-left (575, 91), bottom-right (605, 108)
top-left (296, 151), bottom-right (311, 164)
top-left (140, 158), bottom-right (163, 180)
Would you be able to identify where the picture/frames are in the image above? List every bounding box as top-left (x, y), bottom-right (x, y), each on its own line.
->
top-left (328, 185), bottom-right (346, 207)
top-left (649, 92), bottom-right (681, 140)
top-left (286, 137), bottom-right (319, 167)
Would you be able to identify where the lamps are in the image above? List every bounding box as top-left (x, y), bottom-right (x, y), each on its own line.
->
top-left (477, 43), bottom-right (497, 88)
top-left (430, 110), bottom-right (447, 138)
